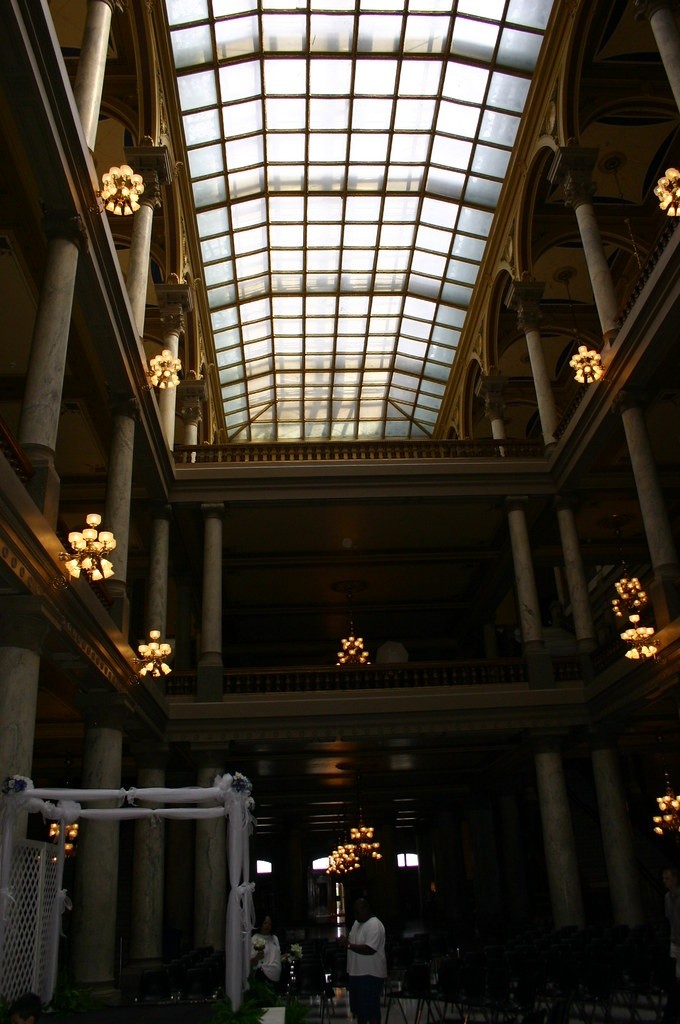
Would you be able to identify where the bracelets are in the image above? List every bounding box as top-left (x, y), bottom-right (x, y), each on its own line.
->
top-left (347, 943), bottom-right (352, 949)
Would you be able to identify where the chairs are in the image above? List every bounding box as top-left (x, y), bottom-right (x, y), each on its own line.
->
top-left (138, 921), bottom-right (679, 1024)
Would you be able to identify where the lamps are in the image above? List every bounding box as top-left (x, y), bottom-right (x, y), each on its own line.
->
top-left (87, 165), bottom-right (146, 216)
top-left (654, 168), bottom-right (680, 218)
top-left (325, 807), bottom-right (383, 876)
top-left (612, 528), bottom-right (660, 664)
top-left (331, 581), bottom-right (370, 666)
top-left (562, 279), bottom-right (603, 384)
top-left (139, 348), bottom-right (182, 392)
top-left (126, 631), bottom-right (171, 686)
top-left (49, 513), bottom-right (117, 591)
top-left (652, 736), bottom-right (680, 834)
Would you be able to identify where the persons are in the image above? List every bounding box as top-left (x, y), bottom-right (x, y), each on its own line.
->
top-left (340, 898), bottom-right (388, 1024)
top-left (248, 914), bottom-right (289, 994)
top-left (662, 866), bottom-right (680, 987)
top-left (7, 992), bottom-right (42, 1024)
